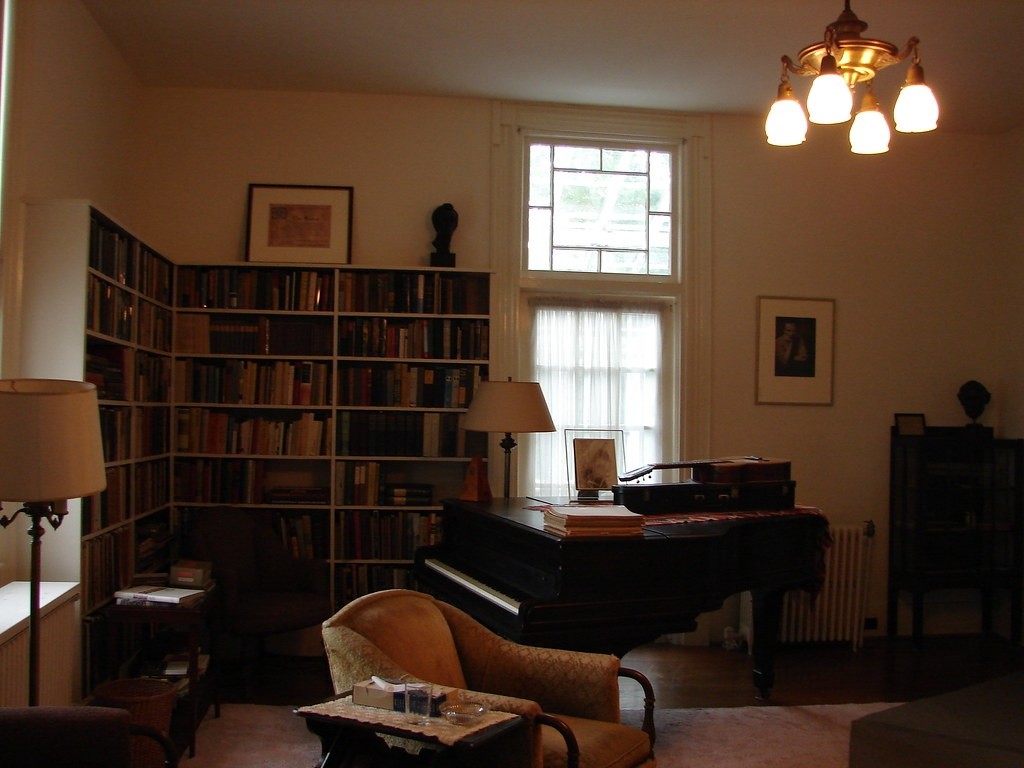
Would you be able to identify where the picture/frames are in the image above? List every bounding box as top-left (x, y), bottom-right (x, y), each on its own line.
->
top-left (895, 413), bottom-right (925, 436)
top-left (563, 427), bottom-right (629, 503)
top-left (247, 183), bottom-right (353, 263)
top-left (754, 296), bottom-right (837, 405)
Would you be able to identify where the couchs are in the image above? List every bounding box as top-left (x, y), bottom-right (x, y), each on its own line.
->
top-left (851, 668), bottom-right (1024, 768)
top-left (322, 589), bottom-right (657, 768)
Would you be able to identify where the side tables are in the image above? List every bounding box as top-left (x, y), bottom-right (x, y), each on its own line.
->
top-left (292, 680), bottom-right (524, 768)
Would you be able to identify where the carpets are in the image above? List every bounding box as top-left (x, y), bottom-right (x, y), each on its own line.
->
top-left (176, 700), bottom-right (905, 768)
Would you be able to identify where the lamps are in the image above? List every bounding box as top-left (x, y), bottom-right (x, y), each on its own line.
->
top-left (0, 378), bottom-right (106, 707)
top-left (460, 377), bottom-right (556, 496)
top-left (765, 0), bottom-right (940, 154)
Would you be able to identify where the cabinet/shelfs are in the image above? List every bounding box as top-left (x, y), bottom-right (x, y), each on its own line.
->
top-left (30, 198), bottom-right (502, 759)
top-left (886, 435), bottom-right (1024, 649)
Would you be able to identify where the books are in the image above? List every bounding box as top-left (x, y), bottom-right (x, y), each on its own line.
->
top-left (543, 504), bottom-right (646, 538)
top-left (82, 216), bottom-right (489, 696)
top-left (352, 675), bottom-right (459, 717)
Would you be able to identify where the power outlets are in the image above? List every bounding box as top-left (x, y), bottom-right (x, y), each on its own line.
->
top-left (865, 617), bottom-right (878, 630)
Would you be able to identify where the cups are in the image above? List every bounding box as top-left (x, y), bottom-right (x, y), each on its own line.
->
top-left (404, 681), bottom-right (432, 725)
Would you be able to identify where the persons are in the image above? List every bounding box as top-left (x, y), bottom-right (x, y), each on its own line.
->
top-left (776, 321), bottom-right (809, 368)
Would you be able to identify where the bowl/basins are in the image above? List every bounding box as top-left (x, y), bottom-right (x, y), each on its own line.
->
top-left (438, 700), bottom-right (490, 725)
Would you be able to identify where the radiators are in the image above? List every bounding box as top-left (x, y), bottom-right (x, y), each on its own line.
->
top-left (740, 520), bottom-right (875, 648)
